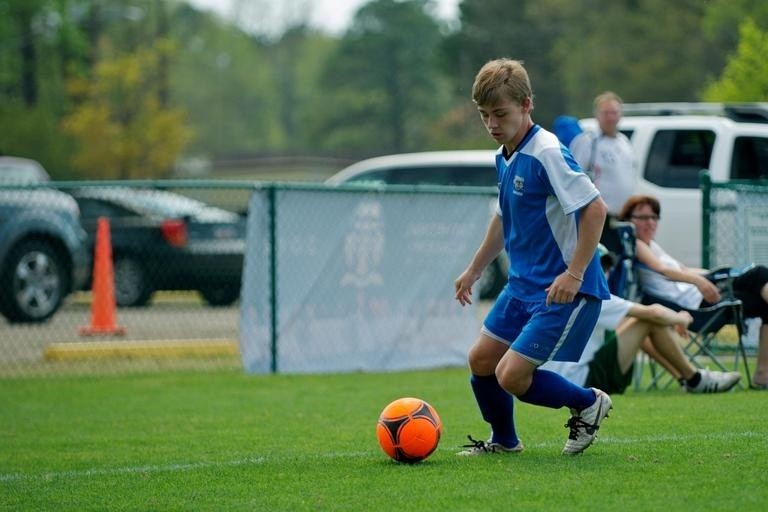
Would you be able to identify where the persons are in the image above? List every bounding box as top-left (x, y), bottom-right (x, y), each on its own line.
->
top-left (622, 194), bottom-right (768, 389)
top-left (560, 242), bottom-right (743, 395)
top-left (454, 57), bottom-right (616, 455)
top-left (569, 91), bottom-right (640, 248)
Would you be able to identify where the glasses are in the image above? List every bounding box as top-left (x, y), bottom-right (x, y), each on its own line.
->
top-left (631, 215), bottom-right (660, 220)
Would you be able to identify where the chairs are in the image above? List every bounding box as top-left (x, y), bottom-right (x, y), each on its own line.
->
top-left (599, 222), bottom-right (755, 393)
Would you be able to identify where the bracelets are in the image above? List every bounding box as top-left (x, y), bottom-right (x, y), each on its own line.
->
top-left (566, 269), bottom-right (584, 282)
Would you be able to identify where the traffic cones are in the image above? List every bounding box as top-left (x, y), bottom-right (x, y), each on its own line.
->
top-left (78, 217), bottom-right (126, 335)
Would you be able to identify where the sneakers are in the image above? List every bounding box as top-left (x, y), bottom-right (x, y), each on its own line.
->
top-left (561, 386), bottom-right (613, 456)
top-left (455, 431), bottom-right (526, 457)
top-left (677, 368), bottom-right (742, 394)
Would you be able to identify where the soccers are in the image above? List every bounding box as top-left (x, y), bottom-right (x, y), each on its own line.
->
top-left (376, 397), bottom-right (442, 463)
